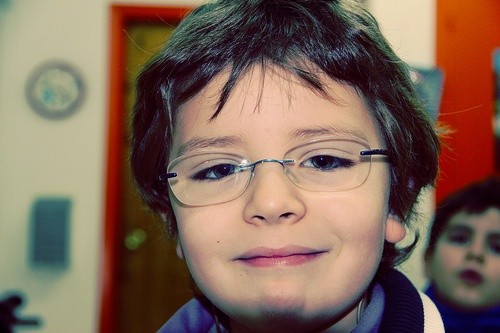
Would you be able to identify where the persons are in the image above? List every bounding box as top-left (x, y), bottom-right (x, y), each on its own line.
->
top-left (131, 0), bottom-right (448, 333)
top-left (423, 173), bottom-right (500, 333)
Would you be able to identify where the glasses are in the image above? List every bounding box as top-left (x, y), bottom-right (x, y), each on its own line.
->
top-left (158, 139), bottom-right (398, 206)
